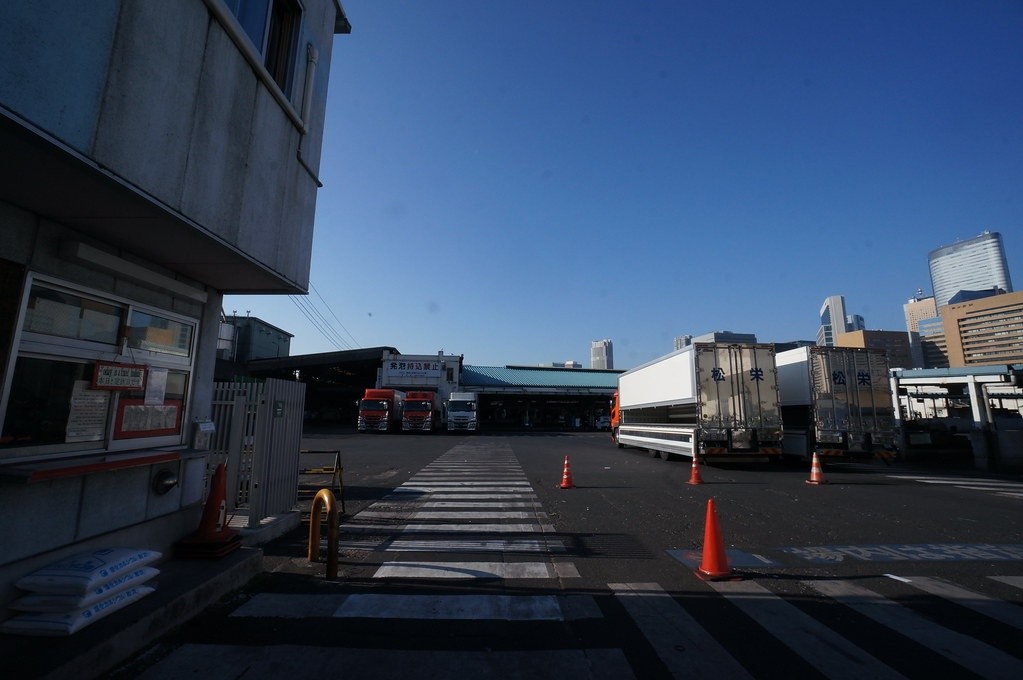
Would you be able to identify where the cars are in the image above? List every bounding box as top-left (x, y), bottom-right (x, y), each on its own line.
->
top-left (594, 416), bottom-right (611, 432)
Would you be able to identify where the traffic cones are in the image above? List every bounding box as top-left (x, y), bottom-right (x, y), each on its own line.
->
top-left (559, 456), bottom-right (575, 489)
top-left (169, 463), bottom-right (243, 560)
top-left (684, 452), bottom-right (704, 485)
top-left (692, 498), bottom-right (744, 582)
top-left (805, 451), bottom-right (828, 486)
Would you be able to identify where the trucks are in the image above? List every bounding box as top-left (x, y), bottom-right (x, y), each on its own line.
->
top-left (356, 388), bottom-right (406, 434)
top-left (444, 392), bottom-right (479, 432)
top-left (399, 391), bottom-right (447, 434)
top-left (609, 340), bottom-right (785, 461)
top-left (775, 346), bottom-right (901, 460)
